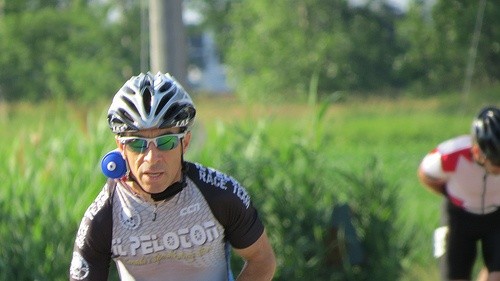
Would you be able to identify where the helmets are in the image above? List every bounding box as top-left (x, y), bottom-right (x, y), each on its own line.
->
top-left (107, 71), bottom-right (197, 134)
top-left (472, 103), bottom-right (500, 168)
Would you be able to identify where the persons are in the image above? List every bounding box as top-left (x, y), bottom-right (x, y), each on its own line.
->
top-left (418, 106), bottom-right (500, 281)
top-left (69, 71), bottom-right (277, 281)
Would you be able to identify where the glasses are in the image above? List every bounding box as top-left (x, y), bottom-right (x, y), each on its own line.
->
top-left (118, 131), bottom-right (185, 153)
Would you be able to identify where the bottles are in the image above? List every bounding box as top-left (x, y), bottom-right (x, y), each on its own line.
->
top-left (101, 148), bottom-right (130, 178)
